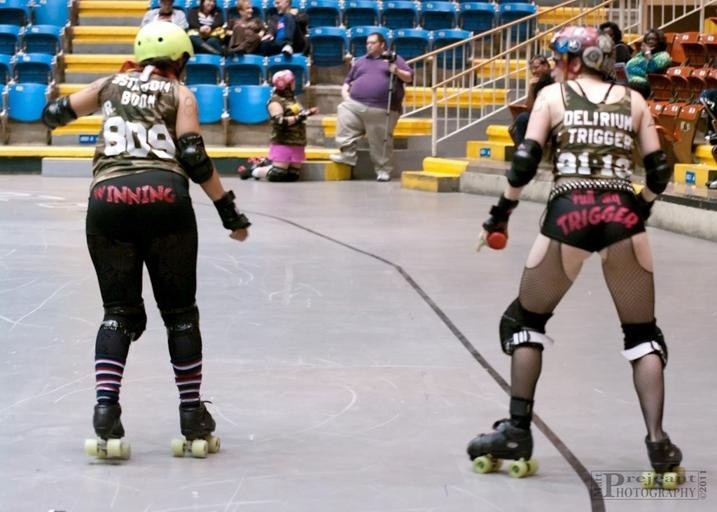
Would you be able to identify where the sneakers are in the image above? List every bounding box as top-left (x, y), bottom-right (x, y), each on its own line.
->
top-left (330, 154), bottom-right (356, 166)
top-left (377, 172), bottom-right (390, 181)
top-left (282, 44), bottom-right (293, 55)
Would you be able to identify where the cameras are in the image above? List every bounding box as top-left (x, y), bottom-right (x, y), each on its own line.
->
top-left (375, 49), bottom-right (397, 63)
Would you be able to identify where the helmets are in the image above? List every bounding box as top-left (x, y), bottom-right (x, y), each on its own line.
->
top-left (134, 21), bottom-right (195, 65)
top-left (549, 25), bottom-right (614, 75)
top-left (273, 69), bottom-right (295, 90)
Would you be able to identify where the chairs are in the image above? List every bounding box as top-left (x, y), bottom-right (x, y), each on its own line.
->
top-left (509, 28), bottom-right (717, 163)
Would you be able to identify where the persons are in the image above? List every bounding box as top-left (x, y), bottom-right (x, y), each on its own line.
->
top-left (466, 25), bottom-right (681, 471)
top-left (42, 22), bottom-right (249, 440)
top-left (260, 0), bottom-right (314, 59)
top-left (506, 21), bottom-right (717, 155)
top-left (225, 0), bottom-right (269, 55)
top-left (236, 69), bottom-right (322, 185)
top-left (186, 0), bottom-right (228, 55)
top-left (140, 0), bottom-right (186, 33)
top-left (327, 29), bottom-right (416, 183)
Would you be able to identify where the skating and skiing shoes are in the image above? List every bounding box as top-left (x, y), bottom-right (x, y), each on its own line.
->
top-left (643, 431), bottom-right (684, 490)
top-left (468, 419), bottom-right (537, 477)
top-left (173, 403), bottom-right (219, 457)
top-left (238, 157), bottom-right (268, 179)
top-left (87, 402), bottom-right (129, 459)
